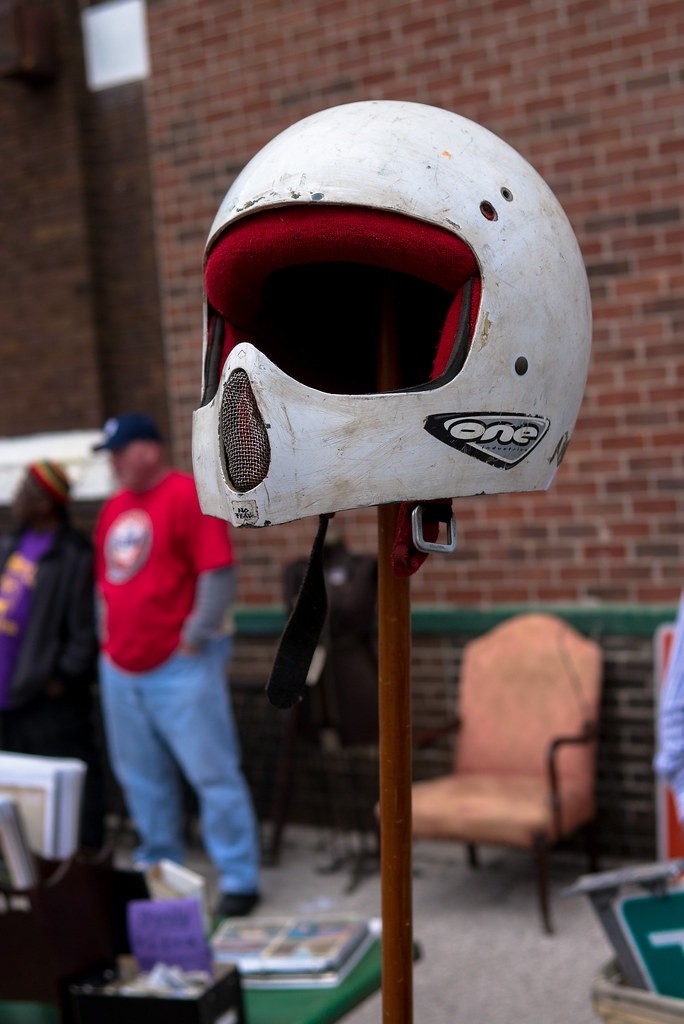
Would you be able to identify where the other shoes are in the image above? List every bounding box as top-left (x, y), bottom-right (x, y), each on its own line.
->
top-left (214, 890), bottom-right (260, 916)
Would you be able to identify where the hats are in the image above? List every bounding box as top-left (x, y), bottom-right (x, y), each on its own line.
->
top-left (28, 460), bottom-right (70, 503)
top-left (93, 412), bottom-right (160, 452)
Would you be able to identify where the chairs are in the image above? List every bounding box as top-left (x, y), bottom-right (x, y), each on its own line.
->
top-left (375, 613), bottom-right (605, 939)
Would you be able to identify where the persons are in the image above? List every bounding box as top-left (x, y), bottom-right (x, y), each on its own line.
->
top-left (93, 414), bottom-right (263, 919)
top-left (0, 457), bottom-right (90, 753)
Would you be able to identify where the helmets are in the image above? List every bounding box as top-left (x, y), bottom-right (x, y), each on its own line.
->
top-left (192, 101), bottom-right (591, 527)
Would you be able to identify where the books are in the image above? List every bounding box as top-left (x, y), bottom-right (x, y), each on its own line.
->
top-left (126, 860), bottom-right (211, 966)
top-left (211, 915), bottom-right (378, 989)
top-left (1, 756), bottom-right (84, 889)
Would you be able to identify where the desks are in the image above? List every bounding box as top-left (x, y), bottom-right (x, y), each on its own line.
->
top-left (0, 919), bottom-right (423, 1024)
